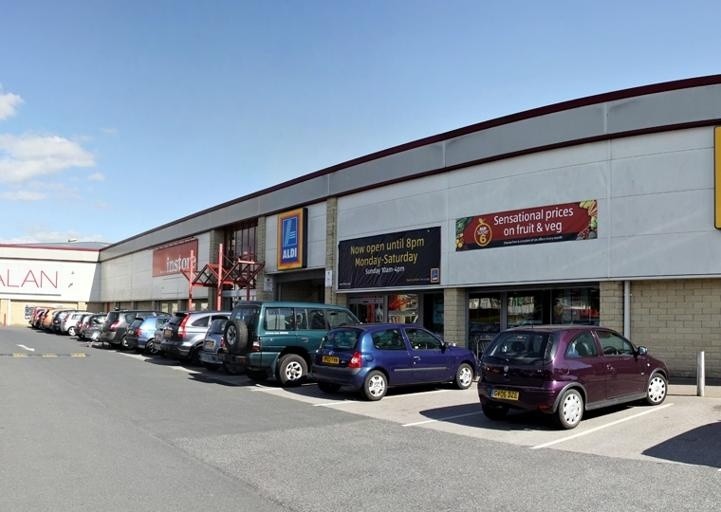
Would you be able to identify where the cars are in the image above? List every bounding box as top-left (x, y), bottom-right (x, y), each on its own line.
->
top-left (311, 322), bottom-right (480, 399)
top-left (478, 322), bottom-right (671, 430)
